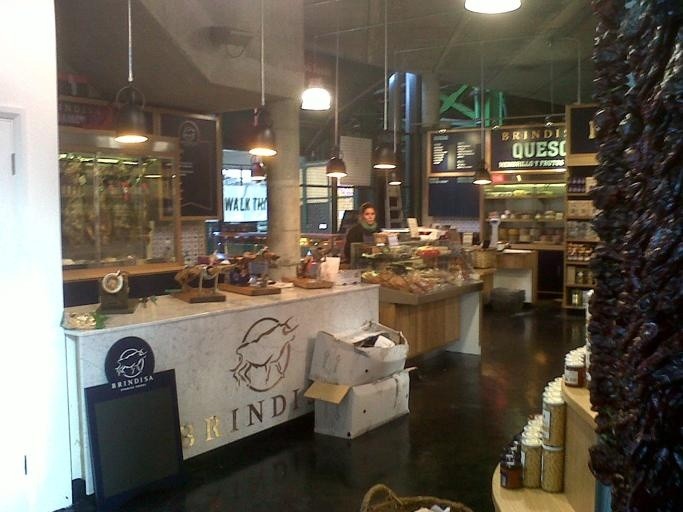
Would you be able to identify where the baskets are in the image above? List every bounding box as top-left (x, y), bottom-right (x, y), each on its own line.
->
top-left (360, 484), bottom-right (470, 512)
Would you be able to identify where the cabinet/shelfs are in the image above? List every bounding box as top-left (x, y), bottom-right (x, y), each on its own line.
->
top-left (478, 183), bottom-right (566, 253)
top-left (561, 157), bottom-right (602, 310)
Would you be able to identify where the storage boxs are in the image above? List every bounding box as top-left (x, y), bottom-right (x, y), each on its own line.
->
top-left (302, 319), bottom-right (418, 440)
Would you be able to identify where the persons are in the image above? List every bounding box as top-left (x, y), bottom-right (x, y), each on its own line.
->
top-left (341, 201), bottom-right (385, 264)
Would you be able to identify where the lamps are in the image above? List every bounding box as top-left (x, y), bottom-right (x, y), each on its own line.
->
top-left (471, 14), bottom-right (491, 187)
top-left (247, 0), bottom-right (276, 156)
top-left (112, 1), bottom-right (148, 144)
top-left (324, 1), bottom-right (395, 179)
top-left (301, 34), bottom-right (330, 109)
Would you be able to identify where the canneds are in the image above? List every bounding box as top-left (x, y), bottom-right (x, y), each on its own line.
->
top-left (568, 243), bottom-right (592, 261)
top-left (500, 346), bottom-right (587, 494)
